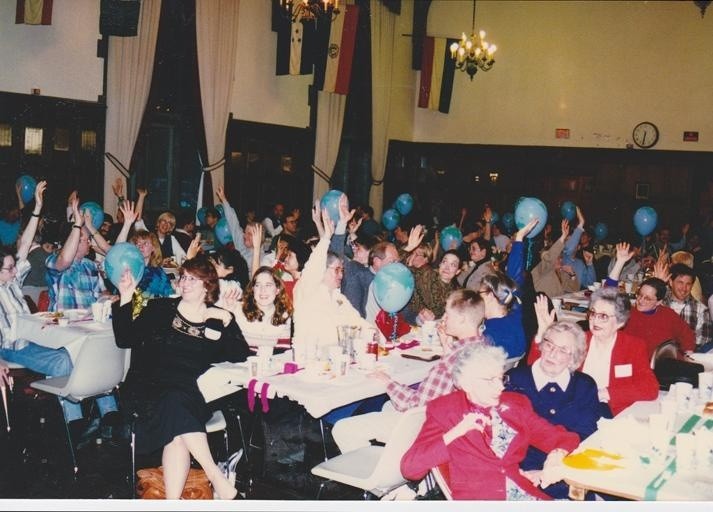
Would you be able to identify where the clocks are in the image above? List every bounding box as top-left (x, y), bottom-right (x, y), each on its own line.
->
top-left (631, 120), bottom-right (659, 147)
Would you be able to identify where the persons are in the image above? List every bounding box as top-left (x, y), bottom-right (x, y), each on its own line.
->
top-left (0, 177), bottom-right (712, 500)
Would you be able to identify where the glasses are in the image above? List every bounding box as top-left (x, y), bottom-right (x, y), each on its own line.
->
top-left (179, 275), bottom-right (204, 286)
top-left (542, 338), bottom-right (570, 359)
top-left (587, 311), bottom-right (616, 322)
top-left (482, 375), bottom-right (513, 386)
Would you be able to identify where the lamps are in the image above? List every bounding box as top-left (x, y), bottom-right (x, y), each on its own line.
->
top-left (285, 0), bottom-right (339, 25)
top-left (449, 0), bottom-right (498, 82)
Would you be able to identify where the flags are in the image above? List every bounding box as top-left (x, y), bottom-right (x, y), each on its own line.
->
top-left (14, 0), bottom-right (52, 24)
top-left (312, 5), bottom-right (359, 95)
top-left (276, 17), bottom-right (314, 76)
top-left (418, 35), bottom-right (461, 114)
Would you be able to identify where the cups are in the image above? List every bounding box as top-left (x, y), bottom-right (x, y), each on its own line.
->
top-left (647, 371), bottom-right (713, 480)
top-left (58, 300), bottom-right (110, 328)
top-left (247, 325), bottom-right (359, 381)
